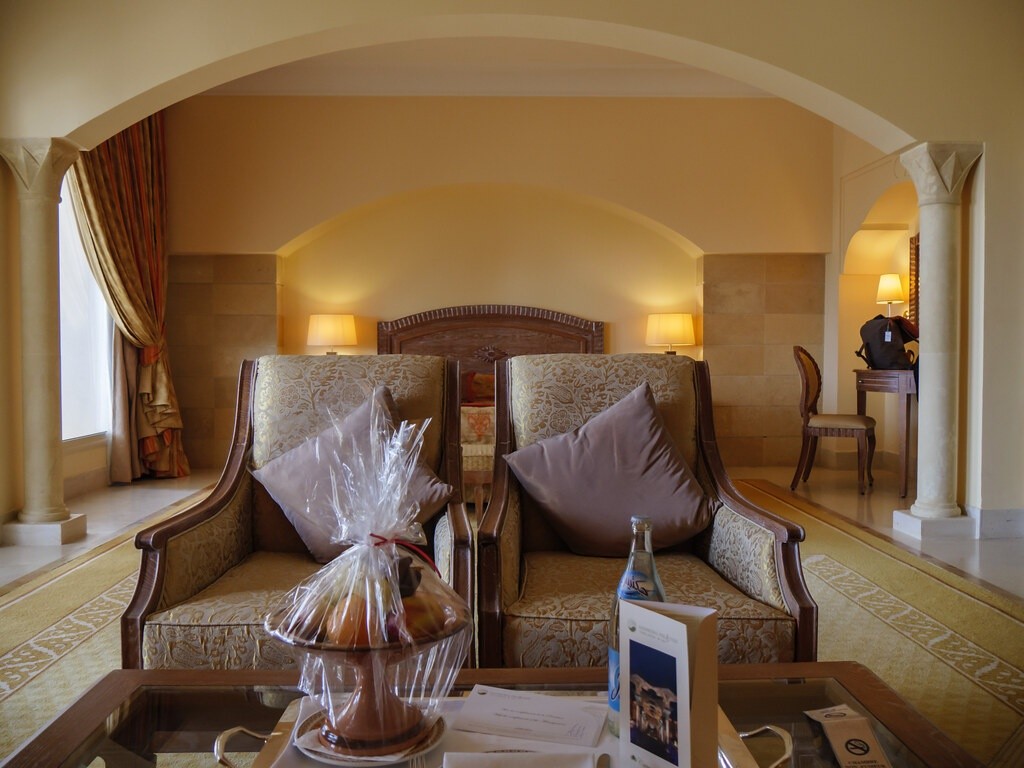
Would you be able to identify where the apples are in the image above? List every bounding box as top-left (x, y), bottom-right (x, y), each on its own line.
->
top-left (295, 572), bottom-right (444, 644)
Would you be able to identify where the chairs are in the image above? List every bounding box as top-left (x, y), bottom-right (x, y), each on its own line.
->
top-left (790, 346), bottom-right (876, 496)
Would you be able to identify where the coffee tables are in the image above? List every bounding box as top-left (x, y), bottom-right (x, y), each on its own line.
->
top-left (5, 663), bottom-right (994, 768)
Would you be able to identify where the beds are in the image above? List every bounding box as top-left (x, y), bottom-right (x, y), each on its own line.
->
top-left (376, 301), bottom-right (604, 505)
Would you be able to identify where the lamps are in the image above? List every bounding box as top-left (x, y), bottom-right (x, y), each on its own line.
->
top-left (646, 314), bottom-right (696, 358)
top-left (307, 313), bottom-right (358, 357)
top-left (875, 273), bottom-right (905, 319)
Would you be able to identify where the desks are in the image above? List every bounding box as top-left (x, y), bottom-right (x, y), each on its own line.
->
top-left (849, 368), bottom-right (916, 500)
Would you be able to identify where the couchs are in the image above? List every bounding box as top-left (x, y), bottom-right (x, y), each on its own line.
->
top-left (118, 353), bottom-right (474, 671)
top-left (473, 350), bottom-right (817, 667)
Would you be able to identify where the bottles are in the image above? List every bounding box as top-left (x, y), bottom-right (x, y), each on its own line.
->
top-left (607, 514), bottom-right (667, 739)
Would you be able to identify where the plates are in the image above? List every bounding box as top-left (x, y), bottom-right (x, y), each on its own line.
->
top-left (293, 701), bottom-right (447, 766)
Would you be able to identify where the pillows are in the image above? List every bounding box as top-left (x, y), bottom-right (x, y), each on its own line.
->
top-left (461, 406), bottom-right (496, 444)
top-left (496, 380), bottom-right (722, 559)
top-left (244, 384), bottom-right (462, 562)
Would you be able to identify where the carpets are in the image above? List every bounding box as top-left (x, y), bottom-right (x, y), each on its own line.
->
top-left (1, 480), bottom-right (1024, 768)
